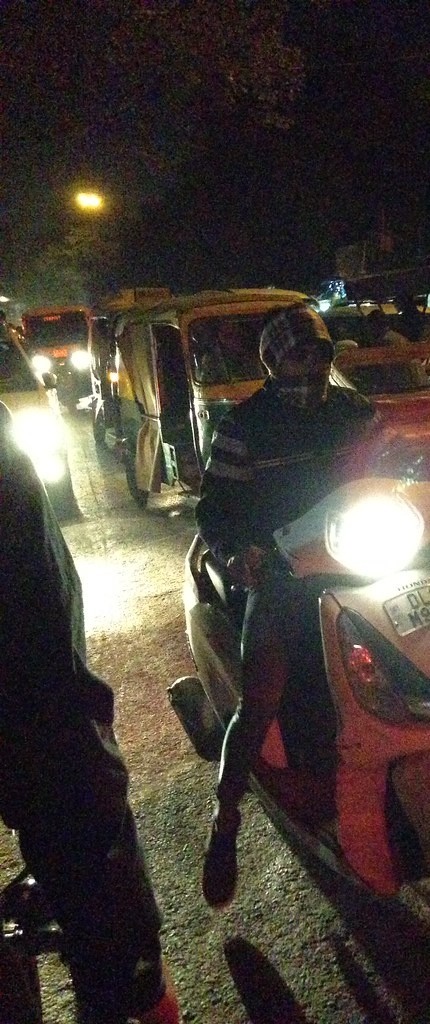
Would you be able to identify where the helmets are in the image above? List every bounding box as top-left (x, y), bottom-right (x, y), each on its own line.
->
top-left (259, 308), bottom-right (335, 407)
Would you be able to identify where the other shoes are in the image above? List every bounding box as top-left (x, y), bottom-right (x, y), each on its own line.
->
top-left (124, 986), bottom-right (183, 1024)
top-left (202, 808), bottom-right (243, 909)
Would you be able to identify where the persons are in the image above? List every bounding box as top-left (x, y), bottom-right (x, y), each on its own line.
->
top-left (391, 281), bottom-right (425, 342)
top-left (201, 315), bottom-right (263, 385)
top-left (365, 309), bottom-right (428, 391)
top-left (195, 301), bottom-right (375, 907)
top-left (0, 446), bottom-right (181, 1024)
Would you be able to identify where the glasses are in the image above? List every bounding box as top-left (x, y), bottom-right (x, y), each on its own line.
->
top-left (284, 345), bottom-right (333, 363)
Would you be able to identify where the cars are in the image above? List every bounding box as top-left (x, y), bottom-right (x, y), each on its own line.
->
top-left (0, 257), bottom-right (429, 520)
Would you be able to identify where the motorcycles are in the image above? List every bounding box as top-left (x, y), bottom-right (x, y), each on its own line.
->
top-left (164, 460), bottom-right (429, 939)
